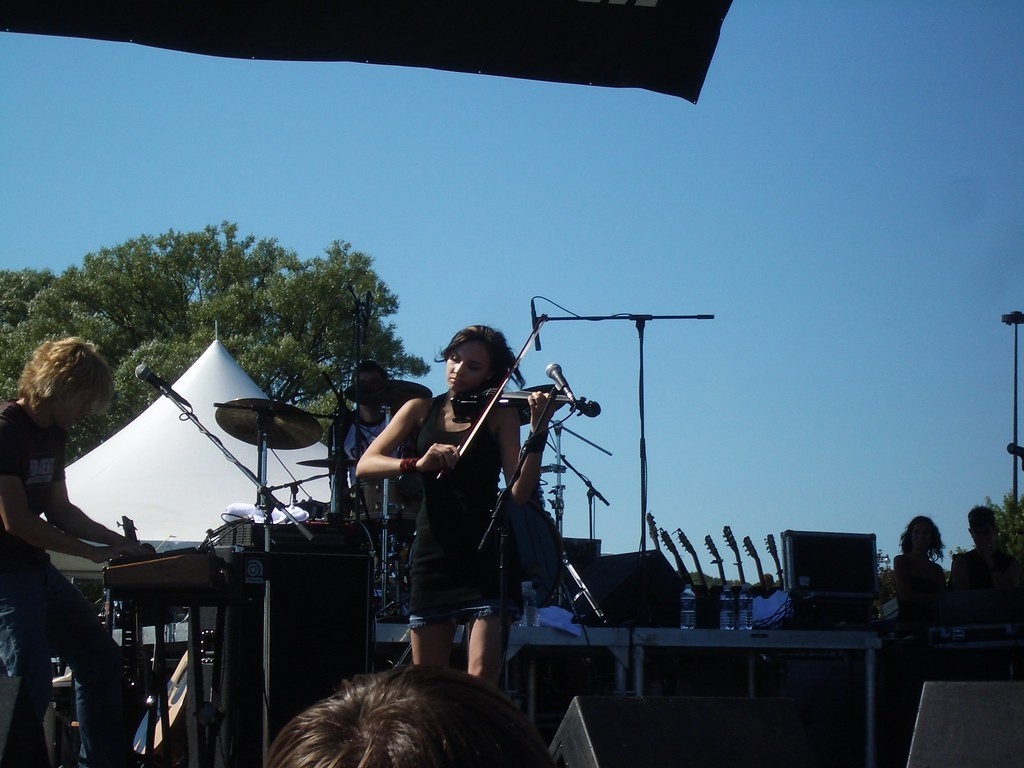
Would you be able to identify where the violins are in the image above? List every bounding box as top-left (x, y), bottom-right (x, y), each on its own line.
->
top-left (450, 385), bottom-right (601, 418)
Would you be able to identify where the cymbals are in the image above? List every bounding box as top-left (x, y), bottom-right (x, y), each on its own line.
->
top-left (343, 380), bottom-right (433, 408)
top-left (296, 456), bottom-right (356, 467)
top-left (216, 398), bottom-right (323, 450)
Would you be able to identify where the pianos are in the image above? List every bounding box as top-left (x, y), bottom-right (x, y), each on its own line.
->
top-left (101, 547), bottom-right (256, 768)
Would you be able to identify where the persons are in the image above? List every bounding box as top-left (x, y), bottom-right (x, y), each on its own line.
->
top-left (949, 504), bottom-right (1024, 591)
top-left (752, 574), bottom-right (791, 627)
top-left (265, 665), bottom-right (557, 768)
top-left (0, 337), bottom-right (155, 768)
top-left (328, 360), bottom-right (423, 517)
top-left (893, 515), bottom-right (948, 639)
top-left (356, 323), bottom-right (555, 688)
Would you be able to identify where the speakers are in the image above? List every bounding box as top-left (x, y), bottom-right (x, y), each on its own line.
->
top-left (904, 680), bottom-right (1024, 768)
top-left (205, 552), bottom-right (376, 768)
top-left (566, 550), bottom-right (711, 628)
top-left (548, 694), bottom-right (818, 768)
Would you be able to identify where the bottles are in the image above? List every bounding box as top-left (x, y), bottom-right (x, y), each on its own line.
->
top-left (737, 583), bottom-right (753, 631)
top-left (679, 583), bottom-right (696, 630)
top-left (517, 581), bottom-right (540, 628)
top-left (719, 585), bottom-right (735, 631)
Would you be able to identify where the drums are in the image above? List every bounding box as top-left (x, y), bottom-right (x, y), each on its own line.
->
top-left (397, 530), bottom-right (417, 592)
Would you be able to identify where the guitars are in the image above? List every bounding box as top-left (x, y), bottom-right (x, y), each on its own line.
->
top-left (133, 649), bottom-right (188, 768)
top-left (646, 513), bottom-right (783, 629)
top-left (540, 464), bottom-right (568, 473)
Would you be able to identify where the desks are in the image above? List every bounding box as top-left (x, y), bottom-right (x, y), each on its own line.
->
top-left (372, 618), bottom-right (882, 767)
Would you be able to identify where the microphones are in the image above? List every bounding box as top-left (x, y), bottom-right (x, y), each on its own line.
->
top-left (134, 365), bottom-right (192, 408)
top-left (545, 363), bottom-right (576, 404)
top-left (530, 300), bottom-right (541, 351)
top-left (361, 290), bottom-right (371, 345)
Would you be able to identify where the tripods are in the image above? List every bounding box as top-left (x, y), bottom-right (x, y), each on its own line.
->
top-left (377, 532), bottom-right (410, 620)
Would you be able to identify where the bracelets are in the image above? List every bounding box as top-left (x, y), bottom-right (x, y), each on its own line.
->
top-left (400, 458), bottom-right (418, 473)
top-left (525, 429), bottom-right (549, 453)
